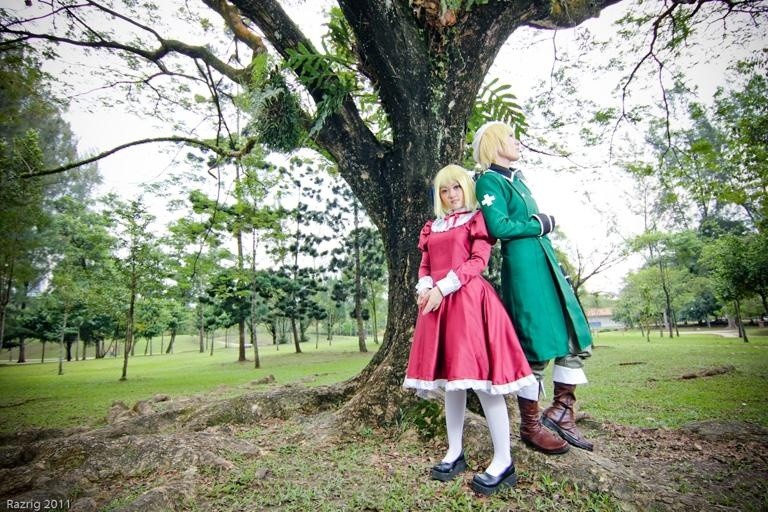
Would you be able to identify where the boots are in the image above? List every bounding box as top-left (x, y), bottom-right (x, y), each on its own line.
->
top-left (539, 376), bottom-right (595, 453)
top-left (515, 380), bottom-right (572, 455)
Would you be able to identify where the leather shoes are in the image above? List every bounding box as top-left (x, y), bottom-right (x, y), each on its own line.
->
top-left (430, 448), bottom-right (469, 482)
top-left (469, 460), bottom-right (518, 496)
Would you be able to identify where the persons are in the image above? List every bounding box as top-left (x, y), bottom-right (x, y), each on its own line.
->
top-left (472, 121), bottom-right (594, 457)
top-left (402, 162), bottom-right (539, 494)
